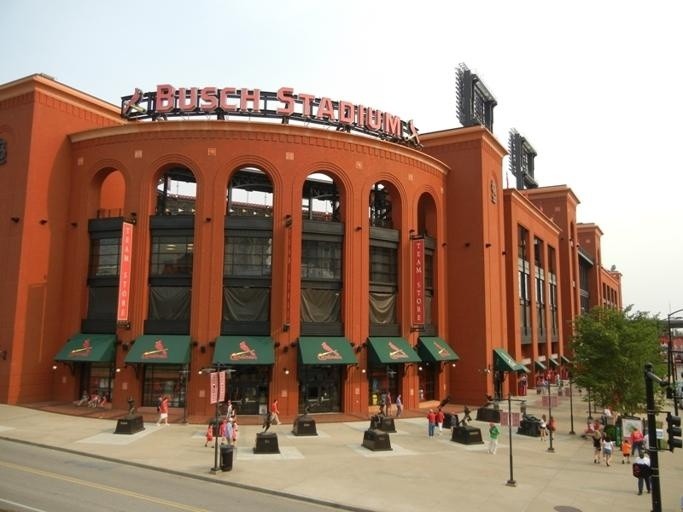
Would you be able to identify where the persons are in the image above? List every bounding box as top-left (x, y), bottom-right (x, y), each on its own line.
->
top-left (629, 425), bottom-right (643, 456)
top-left (436, 408), bottom-right (444, 431)
top-left (385, 391), bottom-right (392, 415)
top-left (88, 392), bottom-right (101, 407)
top-left (592, 425), bottom-right (600, 439)
top-left (537, 414), bottom-right (547, 442)
top-left (426, 409), bottom-right (435, 436)
top-left (78, 390), bottom-right (89, 407)
top-left (218, 415), bottom-right (238, 447)
top-left (204, 423), bottom-right (215, 448)
top-left (620, 439), bottom-right (632, 465)
top-left (156, 397), bottom-right (168, 426)
top-left (633, 449), bottom-right (650, 495)
top-left (397, 394), bottom-right (403, 415)
top-left (516, 370), bottom-right (561, 394)
top-left (489, 422), bottom-right (499, 449)
top-left (546, 416), bottom-right (556, 440)
top-left (100, 395), bottom-right (108, 408)
top-left (593, 435), bottom-right (602, 464)
top-left (270, 399), bottom-right (282, 426)
top-left (226, 401), bottom-right (232, 417)
top-left (157, 395), bottom-right (162, 407)
top-left (602, 436), bottom-right (614, 466)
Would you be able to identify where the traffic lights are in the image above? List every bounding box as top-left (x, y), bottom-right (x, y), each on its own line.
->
top-left (665, 411), bottom-right (682, 452)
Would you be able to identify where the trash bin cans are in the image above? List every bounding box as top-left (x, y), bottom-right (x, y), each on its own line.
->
top-left (219, 445), bottom-right (234, 471)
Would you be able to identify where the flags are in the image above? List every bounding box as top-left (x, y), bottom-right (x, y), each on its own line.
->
top-left (218, 370), bottom-right (226, 403)
top-left (210, 371), bottom-right (217, 404)
